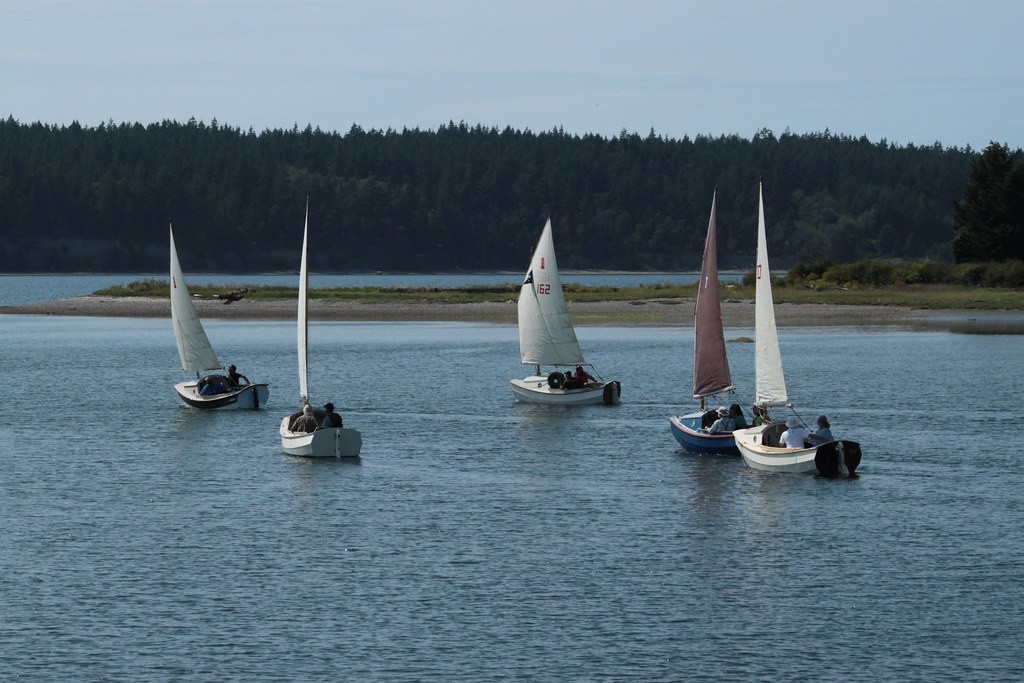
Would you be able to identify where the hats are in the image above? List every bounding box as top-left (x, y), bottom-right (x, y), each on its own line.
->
top-left (786, 417), bottom-right (799, 428)
top-left (565, 371), bottom-right (571, 375)
top-left (323, 403), bottom-right (335, 408)
top-left (204, 376), bottom-right (212, 381)
top-left (751, 405), bottom-right (758, 409)
top-left (715, 406), bottom-right (729, 416)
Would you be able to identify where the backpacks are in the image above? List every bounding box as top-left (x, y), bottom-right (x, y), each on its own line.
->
top-left (328, 413), bottom-right (342, 427)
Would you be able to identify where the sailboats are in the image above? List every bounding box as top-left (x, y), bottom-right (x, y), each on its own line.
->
top-left (669, 189), bottom-right (754, 458)
top-left (731, 181), bottom-right (862, 479)
top-left (278, 191), bottom-right (364, 458)
top-left (509, 216), bottom-right (622, 405)
top-left (167, 221), bottom-right (270, 412)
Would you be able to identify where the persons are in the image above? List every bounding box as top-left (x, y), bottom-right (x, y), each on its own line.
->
top-left (705, 404), bottom-right (771, 435)
top-left (804, 415), bottom-right (834, 449)
top-left (290, 403), bottom-right (326, 433)
top-left (321, 403), bottom-right (342, 428)
top-left (561, 365), bottom-right (598, 390)
top-left (778, 416), bottom-right (810, 450)
top-left (199, 364), bottom-right (250, 395)
